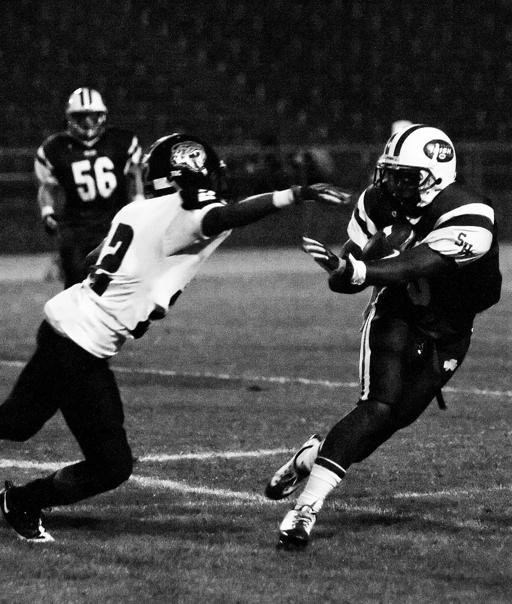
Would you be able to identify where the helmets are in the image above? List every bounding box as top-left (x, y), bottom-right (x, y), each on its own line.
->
top-left (64, 87), bottom-right (108, 148)
top-left (373, 124), bottom-right (457, 207)
top-left (142, 131), bottom-right (228, 199)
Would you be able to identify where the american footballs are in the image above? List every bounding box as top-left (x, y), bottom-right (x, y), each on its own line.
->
top-left (362, 223), bottom-right (416, 262)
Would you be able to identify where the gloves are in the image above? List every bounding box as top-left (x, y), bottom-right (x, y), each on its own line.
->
top-left (41, 214), bottom-right (58, 237)
top-left (301, 235), bottom-right (346, 282)
top-left (301, 183), bottom-right (352, 204)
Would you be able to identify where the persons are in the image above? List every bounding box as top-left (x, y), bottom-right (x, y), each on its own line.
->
top-left (34, 86), bottom-right (144, 288)
top-left (1, 133), bottom-right (353, 545)
top-left (265, 120), bottom-right (502, 550)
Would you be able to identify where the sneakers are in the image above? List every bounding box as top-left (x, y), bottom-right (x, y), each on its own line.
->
top-left (0, 480), bottom-right (56, 543)
top-left (265, 434), bottom-right (322, 499)
top-left (278, 505), bottom-right (317, 546)
